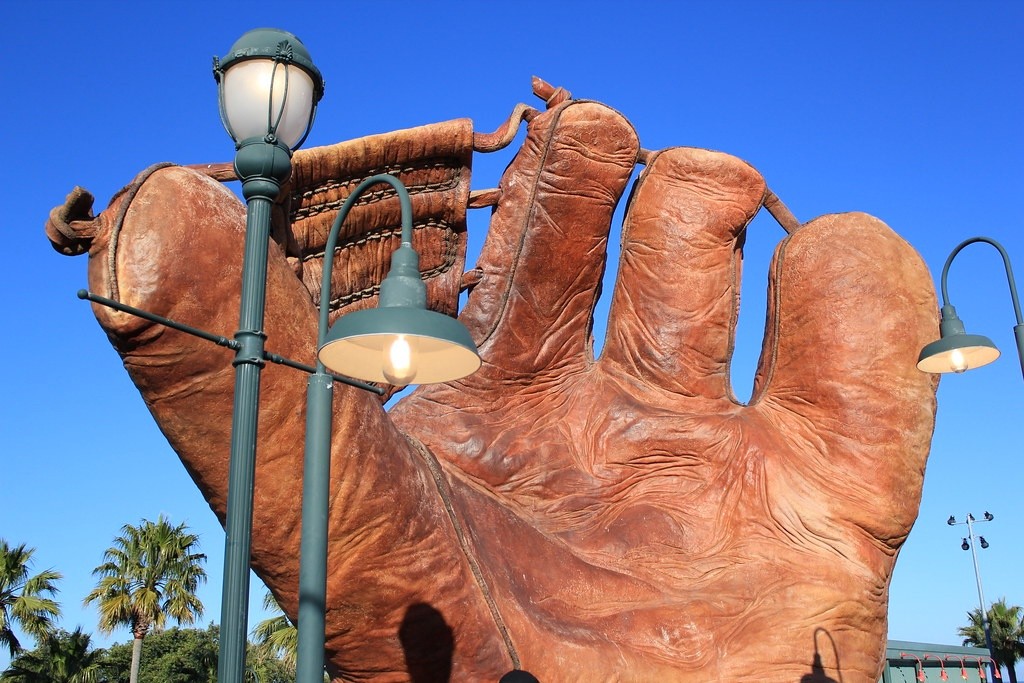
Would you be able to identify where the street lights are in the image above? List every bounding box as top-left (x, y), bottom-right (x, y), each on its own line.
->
top-left (212, 30), bottom-right (328, 683)
top-left (293, 172), bottom-right (480, 683)
top-left (947, 510), bottom-right (1003, 683)
top-left (912, 235), bottom-right (1024, 376)
